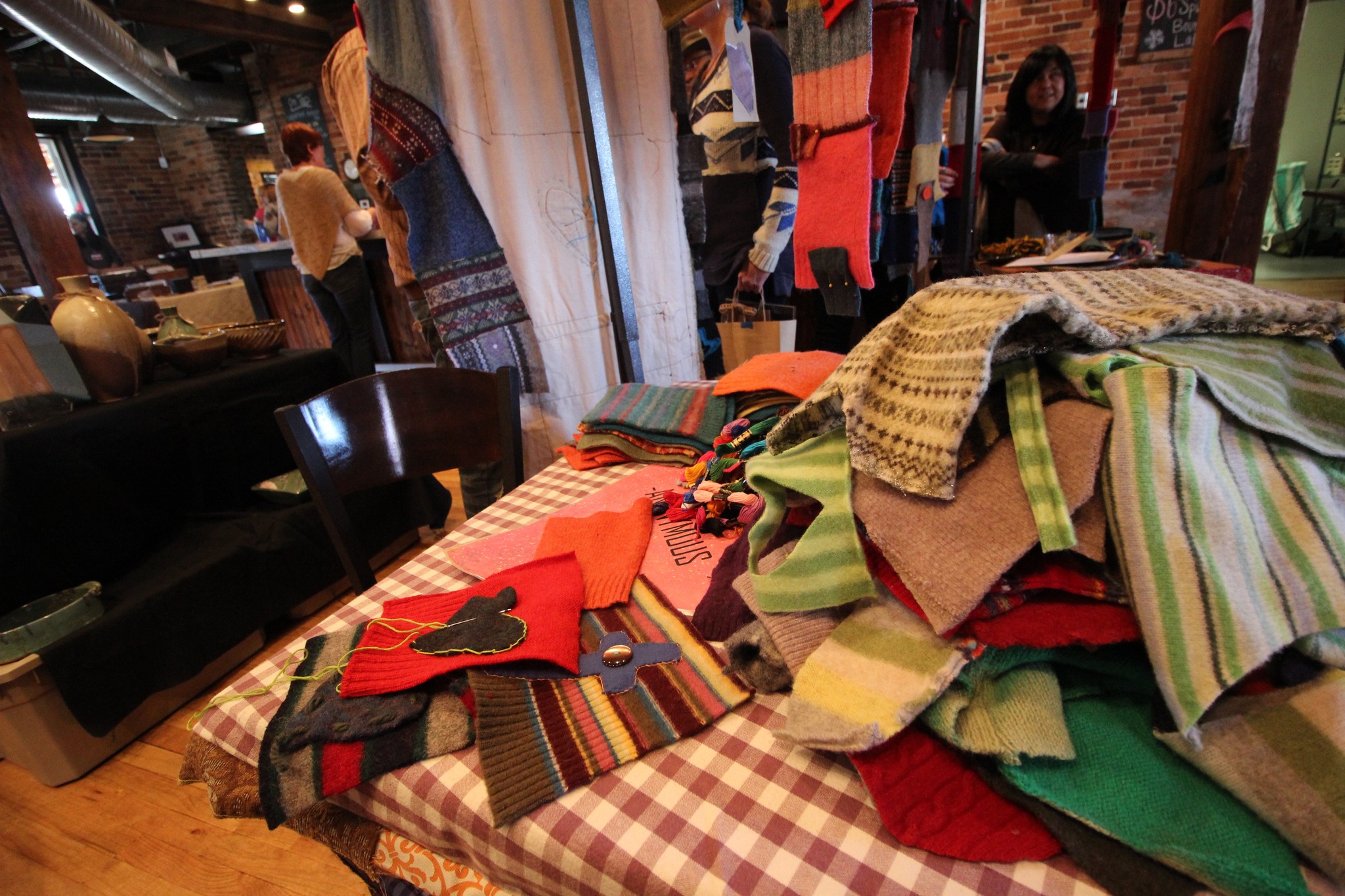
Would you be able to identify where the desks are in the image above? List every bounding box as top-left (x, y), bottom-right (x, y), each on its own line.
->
top-left (117, 279), bottom-right (255, 326)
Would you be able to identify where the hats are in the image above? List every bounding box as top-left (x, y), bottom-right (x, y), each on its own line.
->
top-left (679, 29), bottom-right (706, 53)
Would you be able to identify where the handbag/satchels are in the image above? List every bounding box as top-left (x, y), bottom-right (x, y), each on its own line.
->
top-left (714, 285), bottom-right (799, 375)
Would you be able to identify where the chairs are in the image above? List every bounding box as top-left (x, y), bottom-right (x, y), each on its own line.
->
top-left (92, 263), bottom-right (191, 300)
top-left (275, 367), bottom-right (526, 598)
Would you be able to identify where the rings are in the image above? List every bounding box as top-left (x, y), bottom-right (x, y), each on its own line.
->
top-left (941, 166), bottom-right (946, 174)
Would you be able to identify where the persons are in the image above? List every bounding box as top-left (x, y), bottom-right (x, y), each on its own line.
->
top-left (69, 213), bottom-right (124, 276)
top-left (970, 42), bottom-right (1090, 276)
top-left (871, 75), bottom-right (961, 314)
top-left (676, 21), bottom-right (715, 109)
top-left (320, 26), bottom-right (444, 369)
top-left (252, 183), bottom-right (279, 243)
top-left (274, 121), bottom-right (396, 376)
top-left (682, 0), bottom-right (804, 373)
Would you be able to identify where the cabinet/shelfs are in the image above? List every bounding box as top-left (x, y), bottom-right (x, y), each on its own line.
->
top-left (1, 321), bottom-right (348, 788)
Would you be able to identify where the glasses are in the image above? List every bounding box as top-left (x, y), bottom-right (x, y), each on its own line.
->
top-left (682, 52), bottom-right (711, 73)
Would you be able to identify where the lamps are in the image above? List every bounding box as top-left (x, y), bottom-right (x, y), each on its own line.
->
top-left (82, 80), bottom-right (133, 142)
